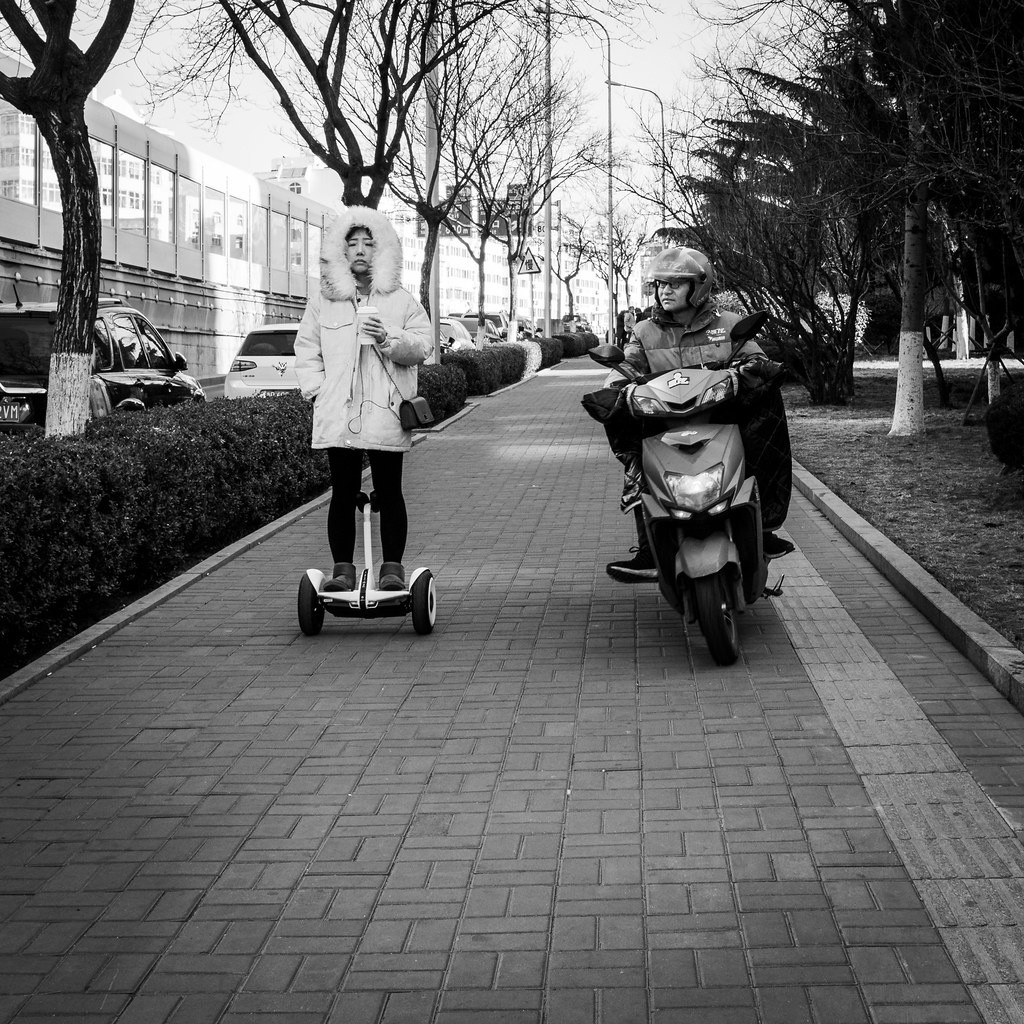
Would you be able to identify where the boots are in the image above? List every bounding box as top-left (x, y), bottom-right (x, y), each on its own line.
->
top-left (323, 562), bottom-right (356, 591)
top-left (379, 562), bottom-right (405, 591)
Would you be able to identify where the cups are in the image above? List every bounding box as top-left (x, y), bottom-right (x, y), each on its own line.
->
top-left (356, 307), bottom-right (380, 346)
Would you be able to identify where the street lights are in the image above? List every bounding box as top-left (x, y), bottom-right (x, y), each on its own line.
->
top-left (605, 79), bottom-right (667, 250)
top-left (534, 5), bottom-right (614, 346)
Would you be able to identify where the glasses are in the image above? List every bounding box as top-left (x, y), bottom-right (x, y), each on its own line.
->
top-left (657, 280), bottom-right (691, 288)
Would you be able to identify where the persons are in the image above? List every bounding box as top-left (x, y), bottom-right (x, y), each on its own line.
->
top-left (292, 204), bottom-right (435, 593)
top-left (605, 248), bottom-right (796, 583)
top-left (624, 306), bottom-right (636, 334)
top-left (635, 308), bottom-right (644, 322)
top-left (616, 311), bottom-right (628, 350)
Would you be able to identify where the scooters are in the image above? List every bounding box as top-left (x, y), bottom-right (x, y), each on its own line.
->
top-left (588, 308), bottom-right (787, 667)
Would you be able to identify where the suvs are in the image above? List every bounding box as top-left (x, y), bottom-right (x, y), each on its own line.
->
top-left (1, 298), bottom-right (207, 437)
top-left (223, 322), bottom-right (301, 399)
top-left (438, 309), bottom-right (592, 355)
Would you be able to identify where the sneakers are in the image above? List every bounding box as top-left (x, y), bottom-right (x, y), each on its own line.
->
top-left (608, 551), bottom-right (657, 585)
top-left (763, 531), bottom-right (795, 558)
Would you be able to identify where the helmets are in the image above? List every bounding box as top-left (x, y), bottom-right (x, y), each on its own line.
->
top-left (654, 248), bottom-right (713, 309)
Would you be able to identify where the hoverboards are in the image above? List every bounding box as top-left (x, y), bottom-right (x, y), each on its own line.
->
top-left (298, 489), bottom-right (436, 636)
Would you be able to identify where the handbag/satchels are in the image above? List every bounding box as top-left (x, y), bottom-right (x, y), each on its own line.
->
top-left (399, 396), bottom-right (435, 431)
